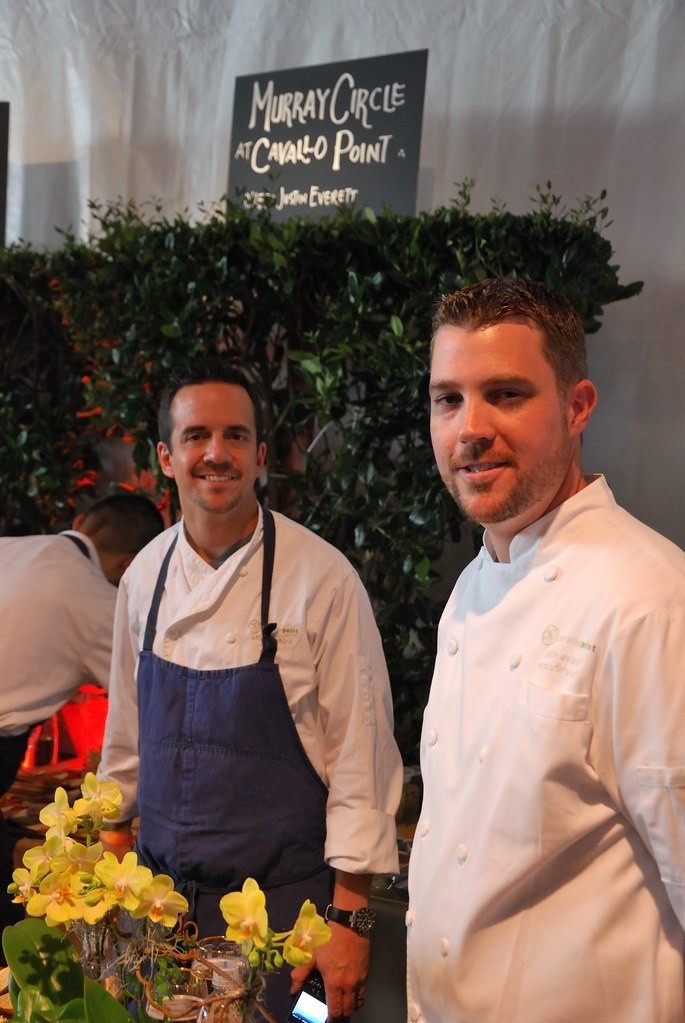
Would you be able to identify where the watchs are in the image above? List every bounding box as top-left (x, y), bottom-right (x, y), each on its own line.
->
top-left (326, 903), bottom-right (376, 935)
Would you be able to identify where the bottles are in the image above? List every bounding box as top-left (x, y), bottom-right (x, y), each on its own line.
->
top-left (195, 962), bottom-right (237, 1023)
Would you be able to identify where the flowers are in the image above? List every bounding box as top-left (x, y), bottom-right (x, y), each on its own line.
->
top-left (3, 771), bottom-right (334, 999)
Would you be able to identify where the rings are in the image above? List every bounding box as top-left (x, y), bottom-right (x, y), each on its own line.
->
top-left (356, 993), bottom-right (367, 998)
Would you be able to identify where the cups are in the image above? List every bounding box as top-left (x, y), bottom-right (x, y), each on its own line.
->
top-left (145, 967), bottom-right (208, 1023)
top-left (190, 936), bottom-right (252, 993)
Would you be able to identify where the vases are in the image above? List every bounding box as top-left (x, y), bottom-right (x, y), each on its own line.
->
top-left (73, 916), bottom-right (124, 999)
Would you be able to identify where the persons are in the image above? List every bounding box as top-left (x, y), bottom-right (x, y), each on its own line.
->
top-left (402, 275), bottom-right (685, 1023)
top-left (93, 365), bottom-right (405, 1023)
top-left (0, 491), bottom-right (164, 961)
